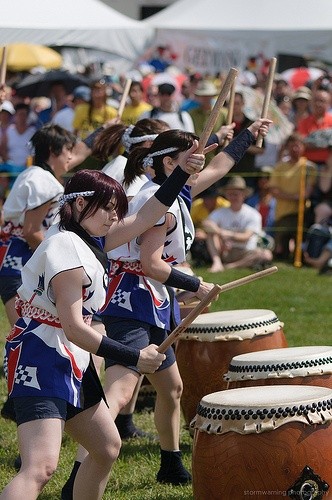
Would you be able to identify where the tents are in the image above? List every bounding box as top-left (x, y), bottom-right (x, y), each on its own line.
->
top-left (0, 0), bottom-right (332, 74)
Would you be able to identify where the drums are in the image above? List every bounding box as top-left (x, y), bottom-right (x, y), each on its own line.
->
top-left (221, 344), bottom-right (332, 390)
top-left (191, 382), bottom-right (332, 500)
top-left (173, 308), bottom-right (288, 428)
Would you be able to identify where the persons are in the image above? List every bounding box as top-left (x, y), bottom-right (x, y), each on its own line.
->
top-left (0, 139), bottom-right (220, 500)
top-left (0, 40), bottom-right (332, 266)
top-left (61, 117), bottom-right (275, 500)
top-left (201, 175), bottom-right (274, 273)
top-left (300, 202), bottom-right (332, 277)
top-left (190, 186), bottom-right (233, 269)
top-left (0, 117), bottom-right (236, 441)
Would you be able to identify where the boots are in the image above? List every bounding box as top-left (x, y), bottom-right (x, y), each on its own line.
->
top-left (156, 446), bottom-right (191, 487)
top-left (61, 461), bottom-right (81, 500)
top-left (115, 412), bottom-right (156, 441)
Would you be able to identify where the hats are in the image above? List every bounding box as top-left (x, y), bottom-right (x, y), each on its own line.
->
top-left (158, 83), bottom-right (175, 96)
top-left (216, 175), bottom-right (255, 198)
top-left (291, 86), bottom-right (311, 102)
top-left (193, 81), bottom-right (219, 97)
